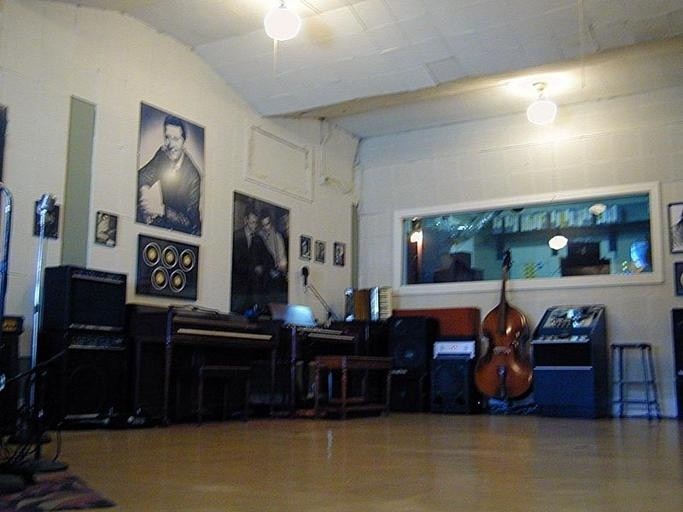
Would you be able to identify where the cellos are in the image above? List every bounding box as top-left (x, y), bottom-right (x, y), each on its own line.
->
top-left (474, 247), bottom-right (532, 401)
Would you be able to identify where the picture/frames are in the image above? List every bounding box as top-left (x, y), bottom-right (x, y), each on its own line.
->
top-left (298, 233), bottom-right (313, 260)
top-left (667, 201), bottom-right (683, 253)
top-left (333, 241), bottom-right (345, 266)
top-left (314, 239), bottom-right (325, 264)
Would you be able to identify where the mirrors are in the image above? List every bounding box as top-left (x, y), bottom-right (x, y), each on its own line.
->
top-left (389, 178), bottom-right (665, 296)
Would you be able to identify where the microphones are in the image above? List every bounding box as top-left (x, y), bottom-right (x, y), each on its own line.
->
top-left (34, 192), bottom-right (55, 214)
top-left (302, 266), bottom-right (309, 285)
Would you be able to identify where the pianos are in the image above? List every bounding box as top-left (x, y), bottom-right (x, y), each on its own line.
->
top-left (127, 301), bottom-right (274, 427)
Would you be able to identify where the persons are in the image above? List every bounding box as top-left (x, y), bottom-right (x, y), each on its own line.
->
top-left (317, 243), bottom-right (324, 261)
top-left (138, 115), bottom-right (202, 235)
top-left (231, 204), bottom-right (288, 313)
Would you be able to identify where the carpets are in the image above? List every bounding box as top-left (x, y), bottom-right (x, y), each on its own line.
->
top-left (0, 448), bottom-right (118, 512)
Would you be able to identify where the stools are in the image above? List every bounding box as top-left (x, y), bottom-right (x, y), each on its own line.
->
top-left (609, 342), bottom-right (662, 422)
top-left (175, 364), bottom-right (252, 426)
top-left (311, 355), bottom-right (394, 420)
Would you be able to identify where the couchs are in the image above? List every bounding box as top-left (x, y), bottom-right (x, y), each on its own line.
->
top-left (289, 312), bottom-right (439, 414)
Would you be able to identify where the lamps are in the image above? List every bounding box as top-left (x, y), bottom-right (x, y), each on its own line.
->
top-left (262, 0), bottom-right (301, 41)
top-left (526, 81), bottom-right (558, 126)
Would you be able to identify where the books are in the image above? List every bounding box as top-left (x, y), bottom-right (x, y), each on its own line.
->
top-left (370, 287), bottom-right (393, 319)
